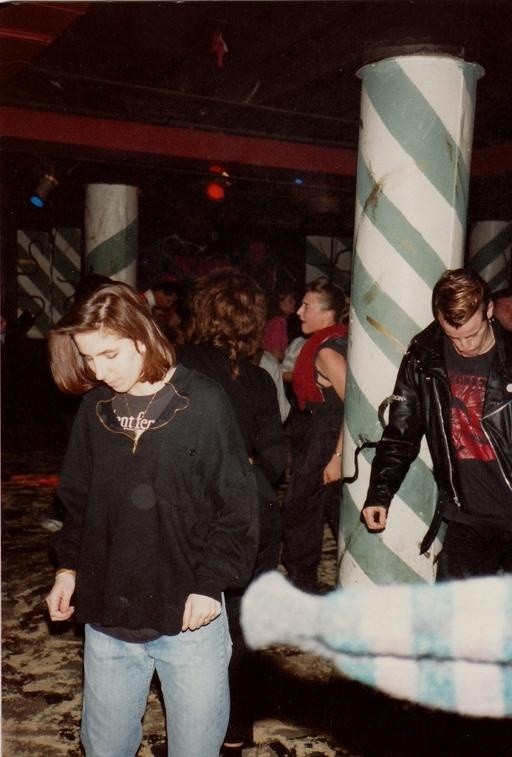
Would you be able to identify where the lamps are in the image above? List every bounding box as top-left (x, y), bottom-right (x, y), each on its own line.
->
top-left (205, 163), bottom-right (236, 202)
top-left (30, 164), bottom-right (63, 208)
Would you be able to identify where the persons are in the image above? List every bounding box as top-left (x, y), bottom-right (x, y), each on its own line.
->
top-left (172, 267), bottom-right (288, 756)
top-left (2, 265), bottom-right (510, 594)
top-left (43, 283), bottom-right (260, 756)
